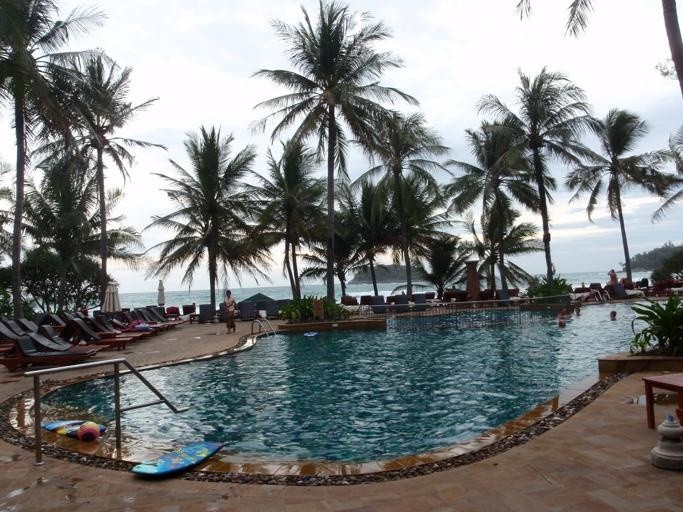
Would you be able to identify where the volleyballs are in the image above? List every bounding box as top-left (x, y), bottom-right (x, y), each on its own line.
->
top-left (78, 422), bottom-right (100, 441)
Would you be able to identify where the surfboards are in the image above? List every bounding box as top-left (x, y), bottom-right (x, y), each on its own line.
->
top-left (131, 442), bottom-right (229, 475)
top-left (43, 420), bottom-right (106, 438)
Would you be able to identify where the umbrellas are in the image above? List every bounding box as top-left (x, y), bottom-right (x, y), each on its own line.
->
top-left (100, 282), bottom-right (122, 325)
top-left (157, 280), bottom-right (165, 306)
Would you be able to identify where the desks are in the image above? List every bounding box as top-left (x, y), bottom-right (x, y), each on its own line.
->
top-left (641, 373), bottom-right (682, 431)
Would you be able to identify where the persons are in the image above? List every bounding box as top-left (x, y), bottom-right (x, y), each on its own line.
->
top-left (223, 290), bottom-right (237, 334)
top-left (575, 307), bottom-right (580, 315)
top-left (610, 311), bottom-right (617, 321)
top-left (558, 306), bottom-right (566, 326)
top-left (608, 270), bottom-right (618, 288)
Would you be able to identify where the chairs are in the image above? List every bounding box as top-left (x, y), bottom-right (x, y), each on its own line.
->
top-left (0, 304), bottom-right (183, 373)
